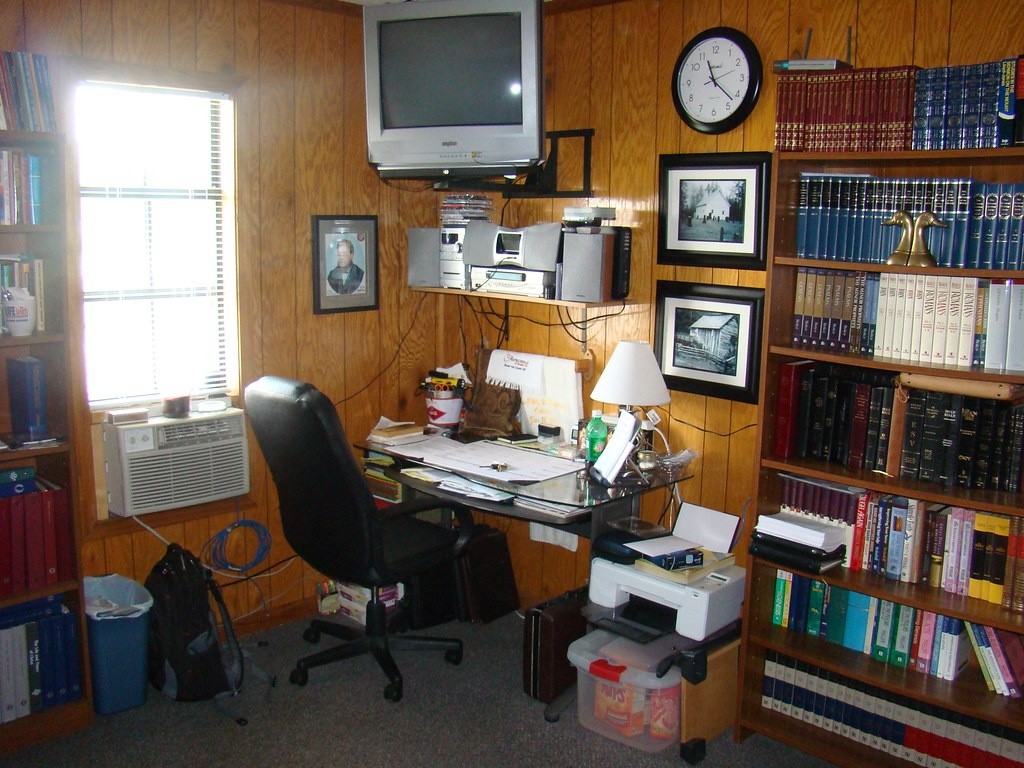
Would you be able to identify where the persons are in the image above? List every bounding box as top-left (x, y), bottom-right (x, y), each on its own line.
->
top-left (327, 239), bottom-right (364, 295)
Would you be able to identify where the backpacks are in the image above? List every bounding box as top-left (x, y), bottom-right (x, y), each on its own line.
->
top-left (139, 543), bottom-right (245, 704)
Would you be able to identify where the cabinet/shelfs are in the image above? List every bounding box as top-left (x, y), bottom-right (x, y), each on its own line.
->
top-left (732, 53), bottom-right (1024, 768)
top-left (0, 49), bottom-right (94, 752)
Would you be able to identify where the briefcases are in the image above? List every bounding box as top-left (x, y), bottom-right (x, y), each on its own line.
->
top-left (524, 584), bottom-right (589, 703)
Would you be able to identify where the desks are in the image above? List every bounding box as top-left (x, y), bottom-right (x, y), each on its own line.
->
top-left (352, 419), bottom-right (695, 723)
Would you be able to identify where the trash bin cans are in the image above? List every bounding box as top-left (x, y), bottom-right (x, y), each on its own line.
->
top-left (84, 573), bottom-right (154, 715)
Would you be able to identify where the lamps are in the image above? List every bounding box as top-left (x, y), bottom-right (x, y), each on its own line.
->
top-left (590, 339), bottom-right (672, 488)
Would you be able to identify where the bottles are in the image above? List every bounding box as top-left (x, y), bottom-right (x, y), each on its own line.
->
top-left (161, 384), bottom-right (190, 418)
top-left (585, 409), bottom-right (608, 462)
top-left (637, 450), bottom-right (656, 471)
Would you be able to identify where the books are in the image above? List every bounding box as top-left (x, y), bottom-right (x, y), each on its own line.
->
top-left (797, 171), bottom-right (1024, 271)
top-left (8, 355), bottom-right (46, 440)
top-left (366, 415), bottom-right (431, 446)
top-left (0, 50), bottom-right (59, 330)
top-left (775, 360), bottom-right (1024, 493)
top-left (772, 568), bottom-right (1024, 698)
top-left (793, 266), bottom-right (1024, 371)
top-left (772, 54), bottom-right (1024, 151)
top-left (760, 650), bottom-right (1024, 768)
top-left (634, 548), bottom-right (735, 584)
top-left (748, 472), bottom-right (1024, 612)
top-left (0, 459), bottom-right (74, 596)
top-left (0, 595), bottom-right (84, 720)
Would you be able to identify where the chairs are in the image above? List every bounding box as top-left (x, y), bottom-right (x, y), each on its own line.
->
top-left (245, 375), bottom-right (475, 703)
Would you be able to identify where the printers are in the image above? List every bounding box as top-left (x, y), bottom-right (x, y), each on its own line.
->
top-left (587, 502), bottom-right (747, 641)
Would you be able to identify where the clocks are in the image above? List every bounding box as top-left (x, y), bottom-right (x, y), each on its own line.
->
top-left (671, 26), bottom-right (763, 135)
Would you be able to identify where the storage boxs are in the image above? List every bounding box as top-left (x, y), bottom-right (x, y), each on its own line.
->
top-left (337, 583), bottom-right (404, 609)
top-left (339, 596), bottom-right (396, 625)
top-left (568, 629), bottom-right (681, 753)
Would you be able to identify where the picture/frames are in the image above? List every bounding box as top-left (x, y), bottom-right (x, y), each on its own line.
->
top-left (657, 151), bottom-right (772, 272)
top-left (653, 279), bottom-right (767, 406)
top-left (311, 214), bottom-right (380, 315)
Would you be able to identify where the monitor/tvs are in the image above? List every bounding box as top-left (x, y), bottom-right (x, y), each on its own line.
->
top-left (363, 0), bottom-right (542, 169)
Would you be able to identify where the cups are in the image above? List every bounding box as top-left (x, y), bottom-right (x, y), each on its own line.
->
top-left (2, 296), bottom-right (37, 337)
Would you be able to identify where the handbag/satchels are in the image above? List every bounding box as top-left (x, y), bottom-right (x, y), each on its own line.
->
top-left (457, 380), bottom-right (520, 439)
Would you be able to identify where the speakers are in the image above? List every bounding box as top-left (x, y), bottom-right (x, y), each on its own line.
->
top-left (561, 233), bottom-right (614, 302)
top-left (408, 227), bottom-right (440, 287)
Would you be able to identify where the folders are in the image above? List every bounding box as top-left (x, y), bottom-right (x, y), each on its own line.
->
top-left (38, 605), bottom-right (83, 708)
top-left (0, 473), bottom-right (73, 593)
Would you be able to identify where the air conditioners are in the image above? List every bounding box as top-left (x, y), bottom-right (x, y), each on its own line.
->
top-left (101, 406), bottom-right (251, 518)
